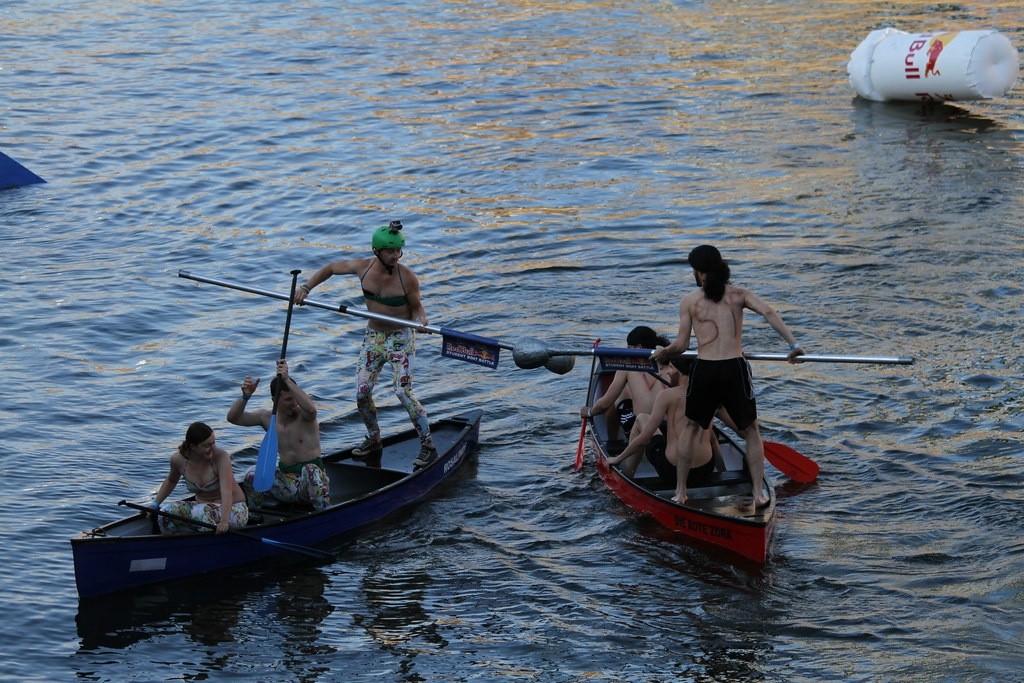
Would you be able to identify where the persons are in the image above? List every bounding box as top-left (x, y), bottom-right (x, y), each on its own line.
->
top-left (606, 362), bottom-right (745, 486)
top-left (226, 359), bottom-right (330, 511)
top-left (140, 423), bottom-right (248, 535)
top-left (581, 326), bottom-right (671, 439)
top-left (649, 245), bottom-right (807, 508)
top-left (290, 226), bottom-right (438, 465)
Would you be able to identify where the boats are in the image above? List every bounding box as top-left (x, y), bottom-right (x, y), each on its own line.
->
top-left (588, 358), bottom-right (778, 565)
top-left (67, 408), bottom-right (484, 601)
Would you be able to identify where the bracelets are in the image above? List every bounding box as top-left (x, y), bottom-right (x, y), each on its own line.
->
top-left (588, 409), bottom-right (591, 417)
top-left (300, 285), bottom-right (311, 293)
top-left (151, 502), bottom-right (159, 509)
top-left (790, 342), bottom-right (800, 350)
top-left (243, 395), bottom-right (248, 400)
top-left (416, 318), bottom-right (424, 322)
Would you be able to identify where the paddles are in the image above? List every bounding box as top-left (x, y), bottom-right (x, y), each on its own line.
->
top-left (651, 368), bottom-right (821, 485)
top-left (574, 337), bottom-right (603, 470)
top-left (118, 499), bottom-right (338, 567)
top-left (252, 268), bottom-right (302, 494)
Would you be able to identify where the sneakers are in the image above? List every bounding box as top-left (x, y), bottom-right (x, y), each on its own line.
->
top-left (413, 445), bottom-right (438, 466)
top-left (352, 434), bottom-right (382, 456)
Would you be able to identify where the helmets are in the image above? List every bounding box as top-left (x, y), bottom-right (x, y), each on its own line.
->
top-left (372, 226), bottom-right (405, 249)
